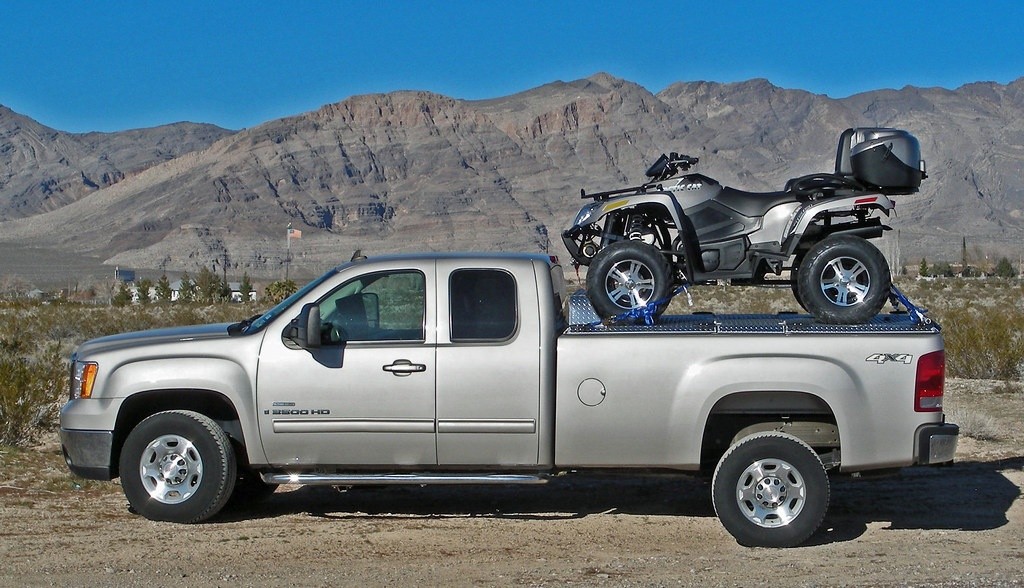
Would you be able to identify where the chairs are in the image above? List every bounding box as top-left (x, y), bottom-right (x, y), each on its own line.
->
top-left (450, 311), bottom-right (480, 338)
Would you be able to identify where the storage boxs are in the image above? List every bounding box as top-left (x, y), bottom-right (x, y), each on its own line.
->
top-left (850, 127), bottom-right (922, 189)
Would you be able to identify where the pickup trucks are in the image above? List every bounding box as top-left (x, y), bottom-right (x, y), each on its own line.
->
top-left (50, 248), bottom-right (962, 547)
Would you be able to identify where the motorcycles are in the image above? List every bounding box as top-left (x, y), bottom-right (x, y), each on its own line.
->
top-left (557, 124), bottom-right (931, 323)
